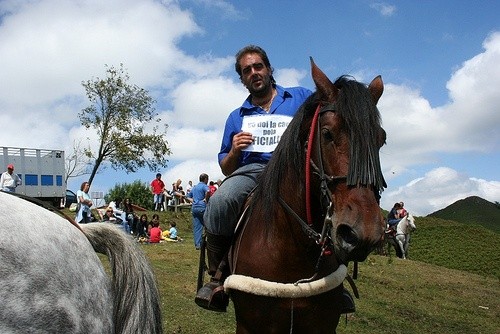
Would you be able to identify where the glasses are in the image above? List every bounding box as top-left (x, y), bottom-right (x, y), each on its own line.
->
top-left (107, 210), bottom-right (113, 213)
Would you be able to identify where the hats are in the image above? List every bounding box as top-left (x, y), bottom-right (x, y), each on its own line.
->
top-left (7, 164), bottom-right (15, 170)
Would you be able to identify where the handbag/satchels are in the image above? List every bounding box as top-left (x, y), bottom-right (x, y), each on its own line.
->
top-left (69, 203), bottom-right (81, 213)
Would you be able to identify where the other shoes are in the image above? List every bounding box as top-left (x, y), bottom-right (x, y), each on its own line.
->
top-left (195, 281), bottom-right (215, 311)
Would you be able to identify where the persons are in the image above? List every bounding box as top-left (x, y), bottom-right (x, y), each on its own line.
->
top-left (0, 164), bottom-right (22, 192)
top-left (196, 45), bottom-right (358, 313)
top-left (77, 173), bottom-right (224, 243)
top-left (384, 202), bottom-right (408, 236)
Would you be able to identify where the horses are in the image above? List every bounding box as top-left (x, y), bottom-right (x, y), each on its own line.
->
top-left (0, 189), bottom-right (162, 334)
top-left (378, 212), bottom-right (417, 259)
top-left (219, 56), bottom-right (386, 334)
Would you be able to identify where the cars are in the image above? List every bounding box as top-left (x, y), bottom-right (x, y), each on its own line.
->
top-left (59, 189), bottom-right (78, 208)
